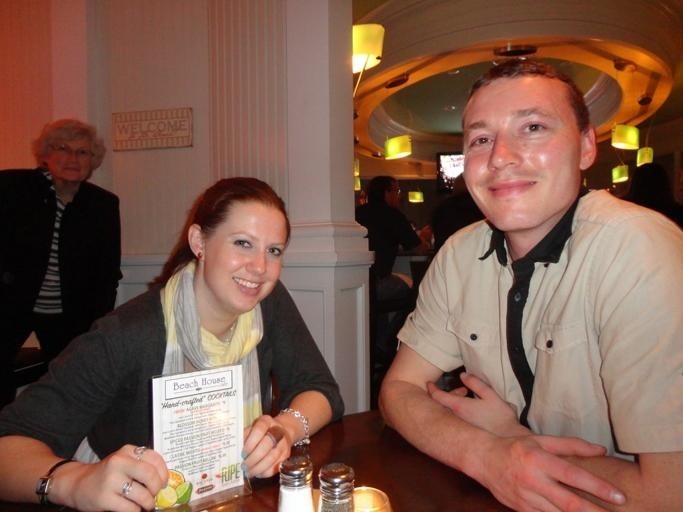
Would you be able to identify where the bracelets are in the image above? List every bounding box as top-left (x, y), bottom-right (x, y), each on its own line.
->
top-left (279, 408), bottom-right (311, 447)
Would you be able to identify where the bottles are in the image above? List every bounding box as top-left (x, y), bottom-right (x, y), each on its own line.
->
top-left (277, 456), bottom-right (354, 512)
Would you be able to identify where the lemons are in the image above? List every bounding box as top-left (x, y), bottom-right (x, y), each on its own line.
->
top-left (154, 486), bottom-right (177, 507)
top-left (175, 483), bottom-right (193, 504)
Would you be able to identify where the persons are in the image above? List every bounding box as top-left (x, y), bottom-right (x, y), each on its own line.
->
top-left (433, 174), bottom-right (481, 240)
top-left (375, 59), bottom-right (683, 512)
top-left (623, 161), bottom-right (683, 232)
top-left (356, 176), bottom-right (418, 322)
top-left (0, 119), bottom-right (120, 391)
top-left (0, 177), bottom-right (346, 512)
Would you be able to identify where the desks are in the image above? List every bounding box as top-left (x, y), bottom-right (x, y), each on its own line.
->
top-left (141, 409), bottom-right (514, 511)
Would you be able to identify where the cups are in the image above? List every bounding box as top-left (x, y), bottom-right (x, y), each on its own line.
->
top-left (354, 486), bottom-right (392, 512)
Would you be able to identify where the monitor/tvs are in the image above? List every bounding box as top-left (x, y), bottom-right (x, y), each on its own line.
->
top-left (436, 152), bottom-right (465, 192)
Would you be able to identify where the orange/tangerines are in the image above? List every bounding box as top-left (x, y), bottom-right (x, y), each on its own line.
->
top-left (166, 470), bottom-right (185, 489)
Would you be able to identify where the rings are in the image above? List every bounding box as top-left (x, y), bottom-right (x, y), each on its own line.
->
top-left (268, 429), bottom-right (284, 445)
top-left (122, 480), bottom-right (134, 495)
top-left (133, 446), bottom-right (148, 457)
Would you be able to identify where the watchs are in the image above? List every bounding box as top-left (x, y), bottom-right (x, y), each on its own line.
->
top-left (36, 459), bottom-right (75, 505)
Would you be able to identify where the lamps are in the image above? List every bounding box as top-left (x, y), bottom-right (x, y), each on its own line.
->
top-left (609, 58), bottom-right (658, 182)
top-left (350, 1), bottom-right (414, 160)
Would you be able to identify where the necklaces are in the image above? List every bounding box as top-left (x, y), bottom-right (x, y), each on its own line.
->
top-left (223, 323), bottom-right (238, 344)
